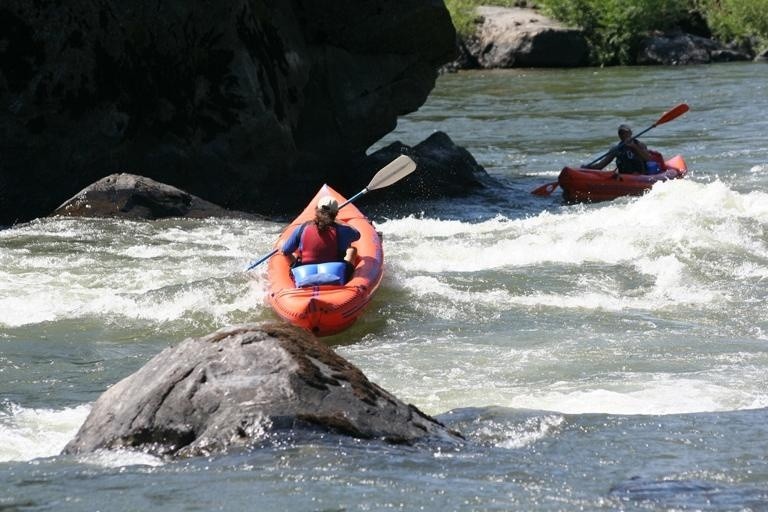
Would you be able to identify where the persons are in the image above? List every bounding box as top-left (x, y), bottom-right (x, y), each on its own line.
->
top-left (580, 124), bottom-right (649, 176)
top-left (277, 196), bottom-right (361, 288)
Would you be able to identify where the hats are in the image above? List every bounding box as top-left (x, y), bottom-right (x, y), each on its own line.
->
top-left (618, 124), bottom-right (631, 134)
top-left (317, 195), bottom-right (339, 212)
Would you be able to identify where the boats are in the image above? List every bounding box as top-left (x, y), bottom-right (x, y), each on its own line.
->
top-left (555, 152), bottom-right (689, 203)
top-left (265, 181), bottom-right (388, 339)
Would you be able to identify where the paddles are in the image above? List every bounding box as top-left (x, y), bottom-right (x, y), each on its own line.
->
top-left (530, 104), bottom-right (689, 196)
top-left (243, 154), bottom-right (417, 271)
